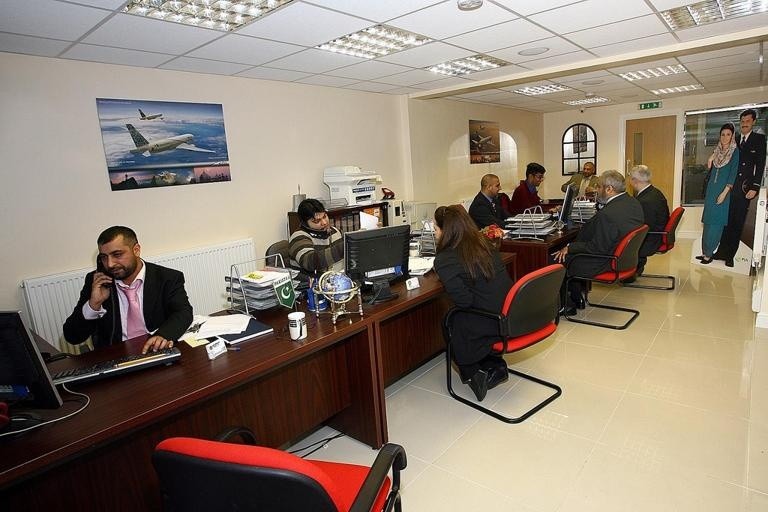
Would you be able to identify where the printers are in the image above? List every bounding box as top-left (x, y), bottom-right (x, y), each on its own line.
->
top-left (324, 165), bottom-right (383, 206)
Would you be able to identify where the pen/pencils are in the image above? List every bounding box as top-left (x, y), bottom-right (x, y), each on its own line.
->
top-left (228, 347), bottom-right (241, 351)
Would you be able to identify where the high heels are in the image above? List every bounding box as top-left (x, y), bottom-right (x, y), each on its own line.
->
top-left (473, 359), bottom-right (509, 401)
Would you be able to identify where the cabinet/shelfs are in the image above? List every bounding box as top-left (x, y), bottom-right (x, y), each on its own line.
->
top-left (288, 200), bottom-right (389, 238)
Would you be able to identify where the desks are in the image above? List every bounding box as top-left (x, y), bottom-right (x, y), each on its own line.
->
top-left (1, 304), bottom-right (385, 511)
top-left (501, 219), bottom-right (593, 293)
top-left (350, 252), bottom-right (517, 445)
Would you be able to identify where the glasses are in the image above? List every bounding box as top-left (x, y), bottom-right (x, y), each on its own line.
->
top-left (536, 175), bottom-right (545, 180)
top-left (275, 317), bottom-right (316, 340)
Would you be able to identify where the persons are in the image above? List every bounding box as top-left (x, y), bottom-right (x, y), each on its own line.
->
top-left (618, 165), bottom-right (669, 283)
top-left (434, 204), bottom-right (513, 401)
top-left (289, 199), bottom-right (382, 293)
top-left (712, 109), bottom-right (766, 267)
top-left (469, 174), bottom-right (509, 229)
top-left (549, 170), bottom-right (644, 316)
top-left (562, 162), bottom-right (598, 196)
top-left (510, 163), bottom-right (561, 216)
top-left (63, 226), bottom-right (193, 355)
top-left (696, 124), bottom-right (740, 264)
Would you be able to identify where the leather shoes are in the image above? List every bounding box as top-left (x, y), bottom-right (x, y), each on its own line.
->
top-left (695, 255), bottom-right (713, 264)
top-left (714, 252), bottom-right (734, 267)
top-left (559, 297), bottom-right (586, 316)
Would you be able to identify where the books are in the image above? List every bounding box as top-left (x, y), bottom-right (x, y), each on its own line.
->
top-left (330, 206), bottom-right (384, 233)
top-left (211, 313), bottom-right (274, 345)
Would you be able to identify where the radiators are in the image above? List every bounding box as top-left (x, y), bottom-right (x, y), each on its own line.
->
top-left (20, 235), bottom-right (258, 353)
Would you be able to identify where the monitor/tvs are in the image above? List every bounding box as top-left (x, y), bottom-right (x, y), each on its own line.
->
top-left (342, 223), bottom-right (412, 305)
top-left (558, 181), bottom-right (579, 222)
top-left (0, 308), bottom-right (64, 409)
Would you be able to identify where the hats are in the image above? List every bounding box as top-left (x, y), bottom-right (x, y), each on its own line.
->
top-left (742, 176), bottom-right (759, 198)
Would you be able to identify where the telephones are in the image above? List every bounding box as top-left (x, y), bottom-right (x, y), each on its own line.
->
top-left (301, 221), bottom-right (328, 239)
top-left (97, 254), bottom-right (113, 288)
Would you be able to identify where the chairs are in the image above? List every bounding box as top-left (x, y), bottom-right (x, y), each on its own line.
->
top-left (150, 423), bottom-right (407, 512)
top-left (618, 207), bottom-right (685, 291)
top-left (441, 262), bottom-right (567, 424)
top-left (564, 224), bottom-right (649, 331)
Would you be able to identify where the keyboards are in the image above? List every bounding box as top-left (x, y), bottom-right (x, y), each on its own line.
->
top-left (51, 344), bottom-right (184, 390)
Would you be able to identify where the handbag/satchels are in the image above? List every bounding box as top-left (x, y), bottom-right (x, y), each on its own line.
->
top-left (701, 176), bottom-right (710, 197)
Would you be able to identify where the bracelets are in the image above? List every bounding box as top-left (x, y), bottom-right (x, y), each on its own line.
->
top-left (567, 243), bottom-right (570, 253)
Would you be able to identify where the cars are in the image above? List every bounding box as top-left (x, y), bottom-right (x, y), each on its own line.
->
top-left (683, 163), bottom-right (709, 203)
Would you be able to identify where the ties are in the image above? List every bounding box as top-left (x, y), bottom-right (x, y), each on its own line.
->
top-left (118, 281), bottom-right (145, 339)
top-left (580, 178), bottom-right (587, 199)
top-left (741, 136), bottom-right (746, 148)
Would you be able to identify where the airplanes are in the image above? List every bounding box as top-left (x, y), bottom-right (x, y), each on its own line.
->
top-left (126, 122), bottom-right (194, 157)
top-left (138, 109), bottom-right (161, 119)
top-left (472, 135), bottom-right (495, 148)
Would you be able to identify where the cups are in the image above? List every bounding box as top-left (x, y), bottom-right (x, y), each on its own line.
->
top-left (306, 288), bottom-right (328, 312)
top-left (409, 242), bottom-right (422, 257)
top-left (287, 311), bottom-right (308, 341)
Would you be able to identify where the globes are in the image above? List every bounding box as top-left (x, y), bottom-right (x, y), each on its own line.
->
top-left (313, 270), bottom-right (363, 325)
